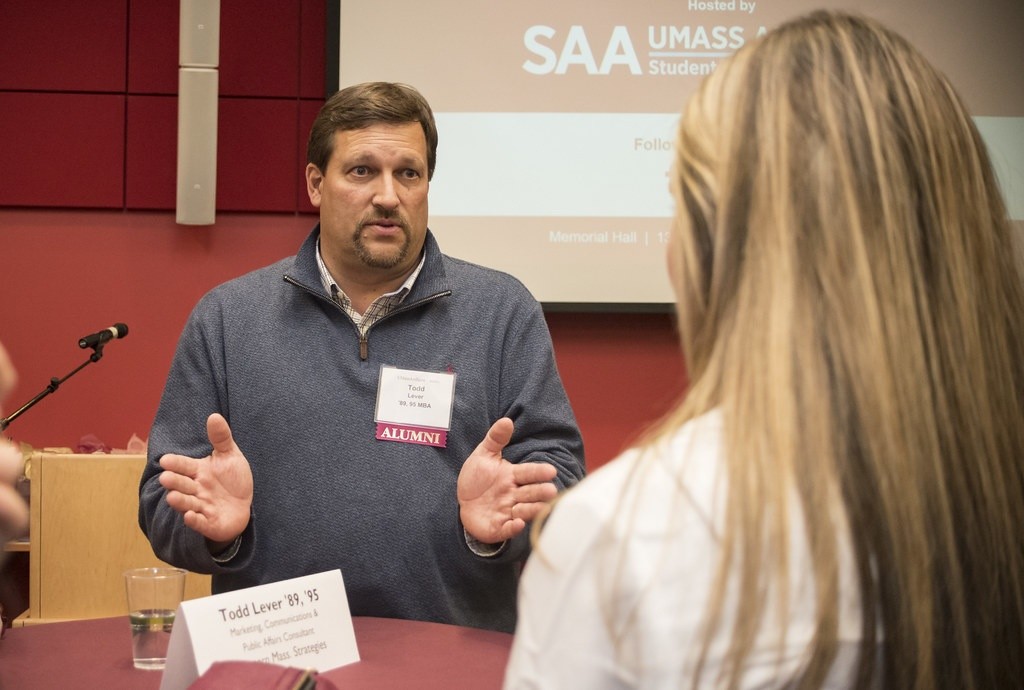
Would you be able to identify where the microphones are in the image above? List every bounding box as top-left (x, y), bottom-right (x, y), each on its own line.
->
top-left (79, 323), bottom-right (128, 349)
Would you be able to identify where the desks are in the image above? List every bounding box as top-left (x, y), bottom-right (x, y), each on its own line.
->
top-left (0, 617), bottom-right (511, 689)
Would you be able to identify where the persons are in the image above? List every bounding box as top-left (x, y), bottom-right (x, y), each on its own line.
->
top-left (138, 81), bottom-right (585, 636)
top-left (506, 10), bottom-right (1024, 690)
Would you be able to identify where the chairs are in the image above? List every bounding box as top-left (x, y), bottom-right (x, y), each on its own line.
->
top-left (10, 452), bottom-right (212, 628)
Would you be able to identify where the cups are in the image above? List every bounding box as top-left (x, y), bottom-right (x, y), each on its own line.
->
top-left (123, 567), bottom-right (187, 670)
